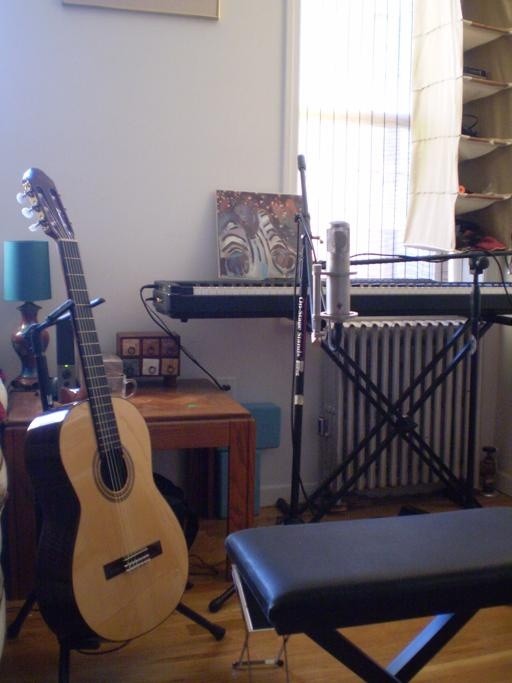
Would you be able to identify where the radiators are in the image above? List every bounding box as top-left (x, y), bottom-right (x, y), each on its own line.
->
top-left (316, 318), bottom-right (482, 513)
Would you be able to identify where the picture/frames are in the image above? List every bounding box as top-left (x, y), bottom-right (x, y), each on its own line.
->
top-left (63, 0), bottom-right (219, 20)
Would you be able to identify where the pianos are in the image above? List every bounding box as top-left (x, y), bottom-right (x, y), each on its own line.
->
top-left (152, 280), bottom-right (510, 322)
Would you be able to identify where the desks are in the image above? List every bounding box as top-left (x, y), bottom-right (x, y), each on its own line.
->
top-left (4, 378), bottom-right (256, 601)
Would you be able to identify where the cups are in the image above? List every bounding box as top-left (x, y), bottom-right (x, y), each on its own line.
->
top-left (105, 371), bottom-right (137, 400)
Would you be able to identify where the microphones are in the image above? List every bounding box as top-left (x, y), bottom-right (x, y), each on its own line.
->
top-left (311, 264), bottom-right (321, 339)
top-left (325, 221), bottom-right (350, 273)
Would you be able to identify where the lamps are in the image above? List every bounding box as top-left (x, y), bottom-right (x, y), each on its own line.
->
top-left (3, 241), bottom-right (52, 389)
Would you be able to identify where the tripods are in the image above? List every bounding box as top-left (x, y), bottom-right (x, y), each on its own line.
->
top-left (7, 298), bottom-right (226, 683)
top-left (208, 153), bottom-right (321, 614)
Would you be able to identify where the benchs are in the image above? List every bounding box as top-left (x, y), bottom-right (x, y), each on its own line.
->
top-left (226, 505), bottom-right (512, 683)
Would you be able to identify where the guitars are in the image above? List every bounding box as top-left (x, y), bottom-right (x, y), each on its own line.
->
top-left (16, 168), bottom-right (189, 645)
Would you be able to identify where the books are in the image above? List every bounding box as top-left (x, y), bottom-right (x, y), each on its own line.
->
top-left (464, 67), bottom-right (487, 80)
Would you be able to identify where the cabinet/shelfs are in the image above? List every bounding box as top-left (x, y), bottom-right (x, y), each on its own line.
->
top-left (405, 0), bottom-right (511, 253)
